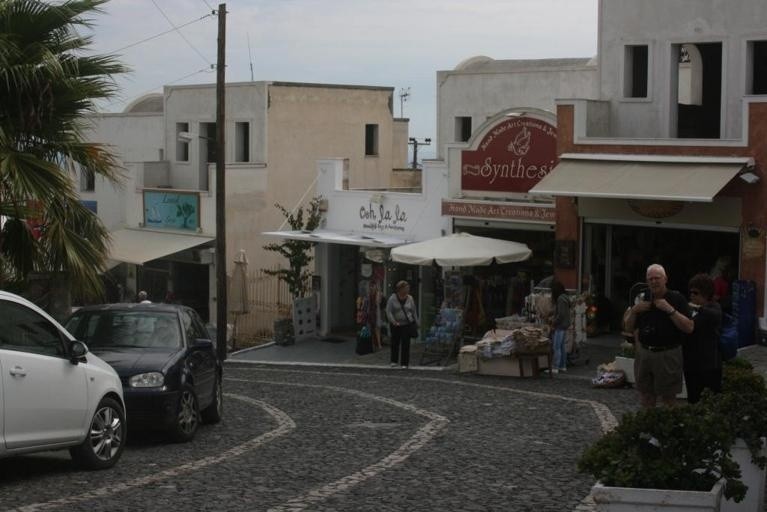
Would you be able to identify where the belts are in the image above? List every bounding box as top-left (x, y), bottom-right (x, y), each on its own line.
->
top-left (640, 344), bottom-right (679, 352)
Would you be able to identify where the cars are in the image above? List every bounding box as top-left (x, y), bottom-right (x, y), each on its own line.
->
top-left (63, 304), bottom-right (219, 435)
top-left (0, 289), bottom-right (126, 469)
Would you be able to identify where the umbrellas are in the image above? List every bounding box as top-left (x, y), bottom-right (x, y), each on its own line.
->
top-left (388, 231), bottom-right (533, 270)
top-left (227, 248), bottom-right (251, 354)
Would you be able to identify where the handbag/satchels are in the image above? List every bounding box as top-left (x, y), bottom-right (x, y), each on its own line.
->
top-left (406, 324), bottom-right (419, 339)
top-left (720, 327), bottom-right (738, 361)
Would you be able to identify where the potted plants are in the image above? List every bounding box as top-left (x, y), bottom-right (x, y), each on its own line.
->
top-left (576, 346), bottom-right (767, 512)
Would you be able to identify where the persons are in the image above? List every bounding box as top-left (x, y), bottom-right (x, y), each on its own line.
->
top-left (385, 281), bottom-right (421, 370)
top-left (620, 260), bottom-right (734, 413)
top-left (116, 283), bottom-right (181, 305)
top-left (544, 280), bottom-right (571, 376)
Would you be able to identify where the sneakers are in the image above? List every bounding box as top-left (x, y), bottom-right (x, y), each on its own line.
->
top-left (547, 367), bottom-right (566, 373)
top-left (390, 363), bottom-right (408, 369)
